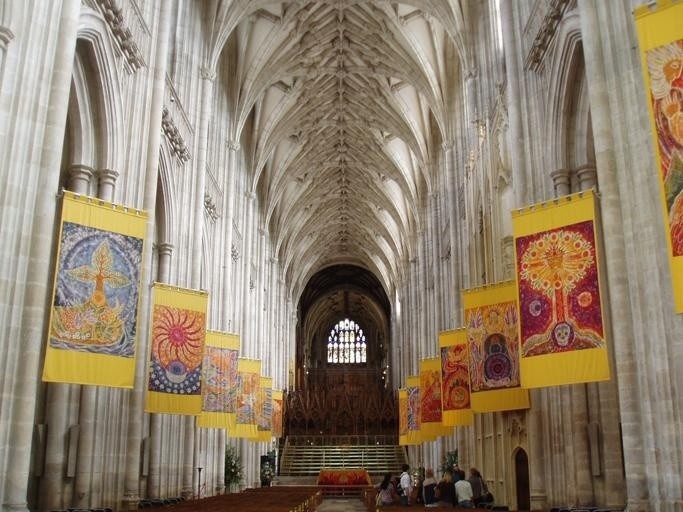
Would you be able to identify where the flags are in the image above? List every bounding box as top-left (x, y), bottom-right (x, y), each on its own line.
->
top-left (511, 189), bottom-right (611, 389)
top-left (143, 281), bottom-right (208, 416)
top-left (461, 278), bottom-right (530, 413)
top-left (229, 357), bottom-right (283, 443)
top-left (399, 328), bottom-right (473, 446)
top-left (632, 0), bottom-right (683, 315)
top-left (196, 330), bottom-right (240, 430)
top-left (41, 190), bottom-right (149, 389)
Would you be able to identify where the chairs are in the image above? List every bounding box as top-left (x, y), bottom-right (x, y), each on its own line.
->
top-left (478, 503), bottom-right (612, 512)
top-left (49, 497), bottom-right (185, 512)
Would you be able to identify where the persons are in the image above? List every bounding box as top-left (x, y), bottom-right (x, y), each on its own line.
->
top-left (377, 464), bottom-right (494, 509)
